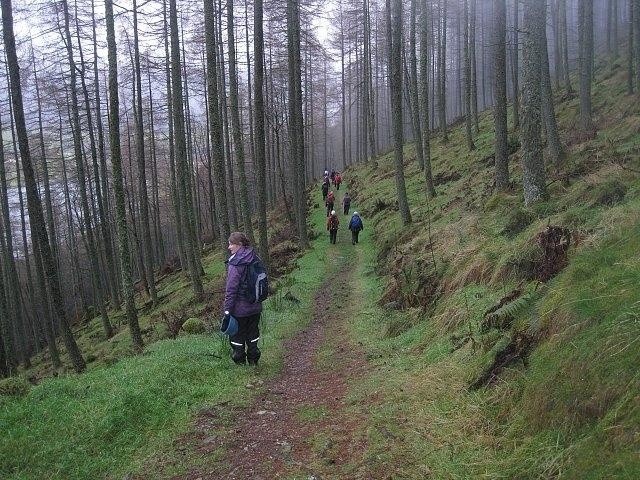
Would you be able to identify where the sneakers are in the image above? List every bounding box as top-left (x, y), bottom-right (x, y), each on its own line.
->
top-left (352, 241), bottom-right (355, 245)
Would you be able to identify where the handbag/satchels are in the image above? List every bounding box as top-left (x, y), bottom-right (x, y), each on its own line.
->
top-left (351, 216), bottom-right (362, 232)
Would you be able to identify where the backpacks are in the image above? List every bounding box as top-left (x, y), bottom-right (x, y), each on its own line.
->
top-left (245, 254), bottom-right (268, 305)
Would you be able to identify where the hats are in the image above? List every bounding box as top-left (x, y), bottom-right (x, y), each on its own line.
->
top-left (353, 211), bottom-right (358, 215)
top-left (331, 210), bottom-right (336, 216)
top-left (220, 314), bottom-right (239, 336)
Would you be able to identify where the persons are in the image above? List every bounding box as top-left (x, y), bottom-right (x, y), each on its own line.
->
top-left (223, 231), bottom-right (268, 366)
top-left (321, 168), bottom-right (364, 247)
